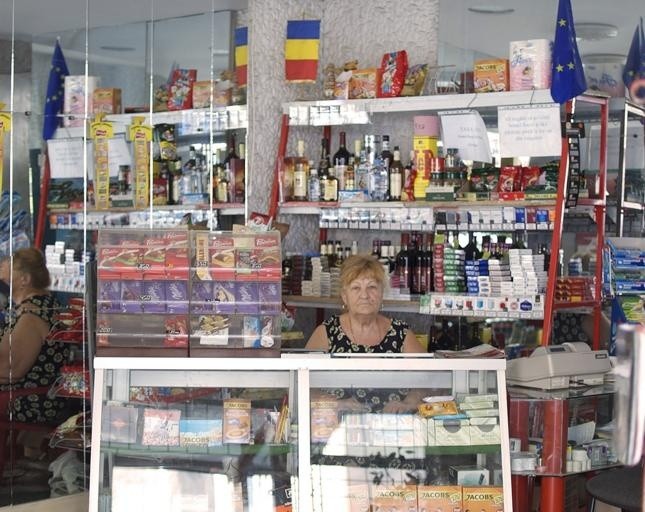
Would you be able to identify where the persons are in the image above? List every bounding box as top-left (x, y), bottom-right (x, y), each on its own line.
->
top-left (304, 254), bottom-right (453, 486)
top-left (0, 244), bottom-right (79, 426)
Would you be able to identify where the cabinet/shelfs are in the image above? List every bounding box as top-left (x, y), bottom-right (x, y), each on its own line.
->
top-left (51, 91), bottom-right (645, 452)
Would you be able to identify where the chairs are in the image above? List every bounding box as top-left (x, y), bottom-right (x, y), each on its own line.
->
top-left (0, 385), bottom-right (77, 478)
top-left (586, 455), bottom-right (644, 511)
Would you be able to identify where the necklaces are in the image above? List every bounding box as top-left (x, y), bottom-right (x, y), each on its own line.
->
top-left (349, 314), bottom-right (361, 344)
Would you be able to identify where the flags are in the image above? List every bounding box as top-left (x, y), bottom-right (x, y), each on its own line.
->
top-left (288, 21), bottom-right (320, 84)
top-left (232, 29), bottom-right (247, 92)
top-left (549, 5), bottom-right (586, 106)
top-left (623, 23), bottom-right (643, 104)
top-left (40, 45), bottom-right (70, 134)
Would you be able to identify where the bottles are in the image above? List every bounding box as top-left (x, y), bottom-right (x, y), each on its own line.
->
top-left (282, 231), bottom-right (514, 297)
top-left (180, 133), bottom-right (466, 203)
